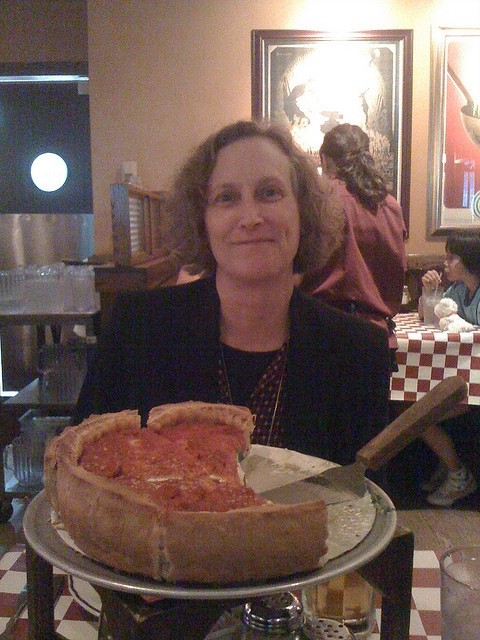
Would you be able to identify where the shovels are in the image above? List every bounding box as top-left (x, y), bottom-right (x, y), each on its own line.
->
top-left (256, 374), bottom-right (469, 507)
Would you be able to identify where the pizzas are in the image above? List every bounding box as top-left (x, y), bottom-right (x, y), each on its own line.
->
top-left (45, 401), bottom-right (330, 587)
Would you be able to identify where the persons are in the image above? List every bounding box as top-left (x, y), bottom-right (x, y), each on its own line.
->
top-left (70, 120), bottom-right (392, 489)
top-left (295, 122), bottom-right (407, 373)
top-left (417, 227), bottom-right (479, 507)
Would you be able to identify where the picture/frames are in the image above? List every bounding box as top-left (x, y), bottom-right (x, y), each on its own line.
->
top-left (249, 27), bottom-right (414, 241)
top-left (425, 23), bottom-right (480, 238)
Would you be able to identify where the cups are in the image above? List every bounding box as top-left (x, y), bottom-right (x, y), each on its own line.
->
top-left (33, 343), bottom-right (88, 404)
top-left (421, 285), bottom-right (445, 326)
top-left (440, 544), bottom-right (480, 640)
top-left (3, 432), bottom-right (46, 487)
top-left (0, 262), bottom-right (98, 316)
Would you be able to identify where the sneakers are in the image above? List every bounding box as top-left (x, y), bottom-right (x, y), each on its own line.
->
top-left (423, 464), bottom-right (451, 493)
top-left (426, 471), bottom-right (478, 508)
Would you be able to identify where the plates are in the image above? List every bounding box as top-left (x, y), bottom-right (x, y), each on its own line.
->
top-left (22, 442), bottom-right (399, 601)
